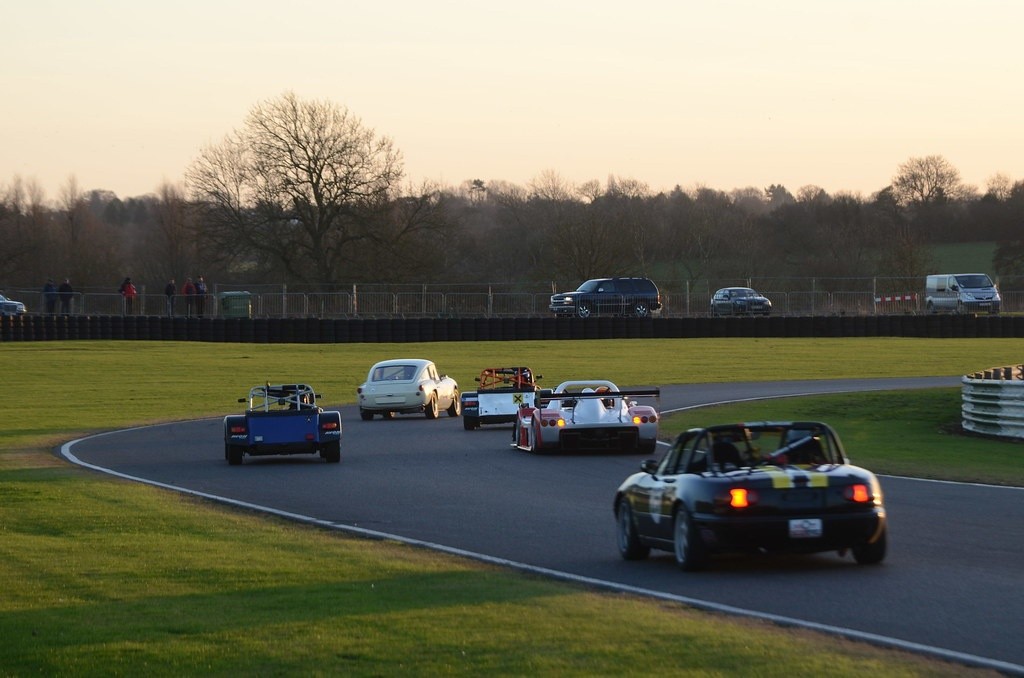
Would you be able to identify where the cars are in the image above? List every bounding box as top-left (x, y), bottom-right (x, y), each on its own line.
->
top-left (0, 295), bottom-right (27, 315)
top-left (460, 366), bottom-right (544, 432)
top-left (614, 422), bottom-right (888, 572)
top-left (711, 287), bottom-right (773, 318)
top-left (224, 382), bottom-right (342, 462)
top-left (357, 355), bottom-right (461, 419)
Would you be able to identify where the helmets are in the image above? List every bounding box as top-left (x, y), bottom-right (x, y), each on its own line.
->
top-left (785, 429), bottom-right (821, 447)
top-left (290, 394), bottom-right (309, 406)
top-left (514, 369), bottom-right (530, 382)
top-left (595, 386), bottom-right (613, 402)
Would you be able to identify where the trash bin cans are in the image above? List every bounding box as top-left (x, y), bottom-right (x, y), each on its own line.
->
top-left (217, 290), bottom-right (252, 320)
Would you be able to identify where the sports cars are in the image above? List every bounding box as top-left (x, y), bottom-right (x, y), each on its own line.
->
top-left (508, 380), bottom-right (662, 453)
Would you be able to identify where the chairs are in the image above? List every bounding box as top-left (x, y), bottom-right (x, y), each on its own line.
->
top-left (289, 394), bottom-right (308, 409)
top-left (705, 443), bottom-right (741, 471)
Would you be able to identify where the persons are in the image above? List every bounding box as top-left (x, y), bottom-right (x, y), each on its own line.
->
top-left (119, 277), bottom-right (136, 315)
top-left (164, 278), bottom-right (177, 317)
top-left (289, 392), bottom-right (311, 409)
top-left (182, 277), bottom-right (196, 319)
top-left (43, 278), bottom-right (56, 317)
top-left (194, 274), bottom-right (208, 318)
top-left (595, 386), bottom-right (613, 408)
top-left (59, 277), bottom-right (73, 315)
top-left (776, 431), bottom-right (821, 466)
top-left (514, 370), bottom-right (531, 387)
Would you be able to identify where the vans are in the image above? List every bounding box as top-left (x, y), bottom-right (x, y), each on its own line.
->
top-left (925, 272), bottom-right (1000, 315)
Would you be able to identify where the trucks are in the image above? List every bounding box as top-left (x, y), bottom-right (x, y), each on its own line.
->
top-left (214, 291), bottom-right (261, 319)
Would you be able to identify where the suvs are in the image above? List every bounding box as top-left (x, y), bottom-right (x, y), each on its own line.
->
top-left (548, 276), bottom-right (660, 317)
